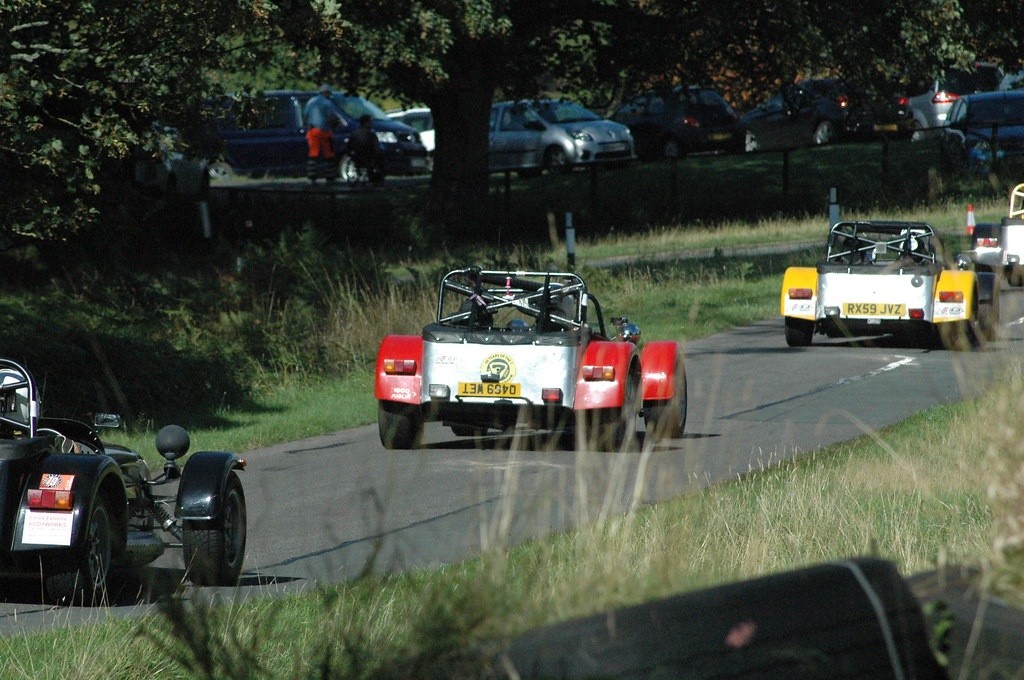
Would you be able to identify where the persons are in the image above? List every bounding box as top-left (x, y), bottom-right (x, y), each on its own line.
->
top-left (0, 366), bottom-right (84, 455)
top-left (302, 83), bottom-right (338, 187)
top-left (899, 227), bottom-right (935, 264)
top-left (339, 113), bottom-right (379, 168)
top-left (534, 283), bottom-right (593, 334)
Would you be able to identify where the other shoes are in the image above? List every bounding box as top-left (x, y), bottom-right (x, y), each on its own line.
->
top-left (309, 179), bottom-right (316, 185)
top-left (326, 178), bottom-right (335, 185)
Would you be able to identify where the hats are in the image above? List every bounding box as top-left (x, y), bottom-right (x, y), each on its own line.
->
top-left (319, 85), bottom-right (334, 94)
top-left (357, 115), bottom-right (372, 124)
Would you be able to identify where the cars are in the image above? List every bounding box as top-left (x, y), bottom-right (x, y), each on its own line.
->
top-left (377, 104), bottom-right (437, 157)
top-left (902, 61), bottom-right (1013, 130)
top-left (943, 86), bottom-right (1024, 159)
top-left (493, 93), bottom-right (638, 177)
top-left (605, 84), bottom-right (748, 165)
top-left (741, 76), bottom-right (915, 151)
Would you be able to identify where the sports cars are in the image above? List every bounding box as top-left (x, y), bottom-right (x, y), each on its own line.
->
top-left (780, 218), bottom-right (1000, 355)
top-left (0, 356), bottom-right (250, 609)
top-left (367, 268), bottom-right (692, 454)
top-left (972, 179), bottom-right (1023, 286)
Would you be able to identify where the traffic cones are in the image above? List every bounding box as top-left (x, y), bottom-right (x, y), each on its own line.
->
top-left (964, 203), bottom-right (978, 236)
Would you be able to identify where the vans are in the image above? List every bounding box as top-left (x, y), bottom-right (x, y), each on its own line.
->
top-left (181, 89), bottom-right (428, 185)
